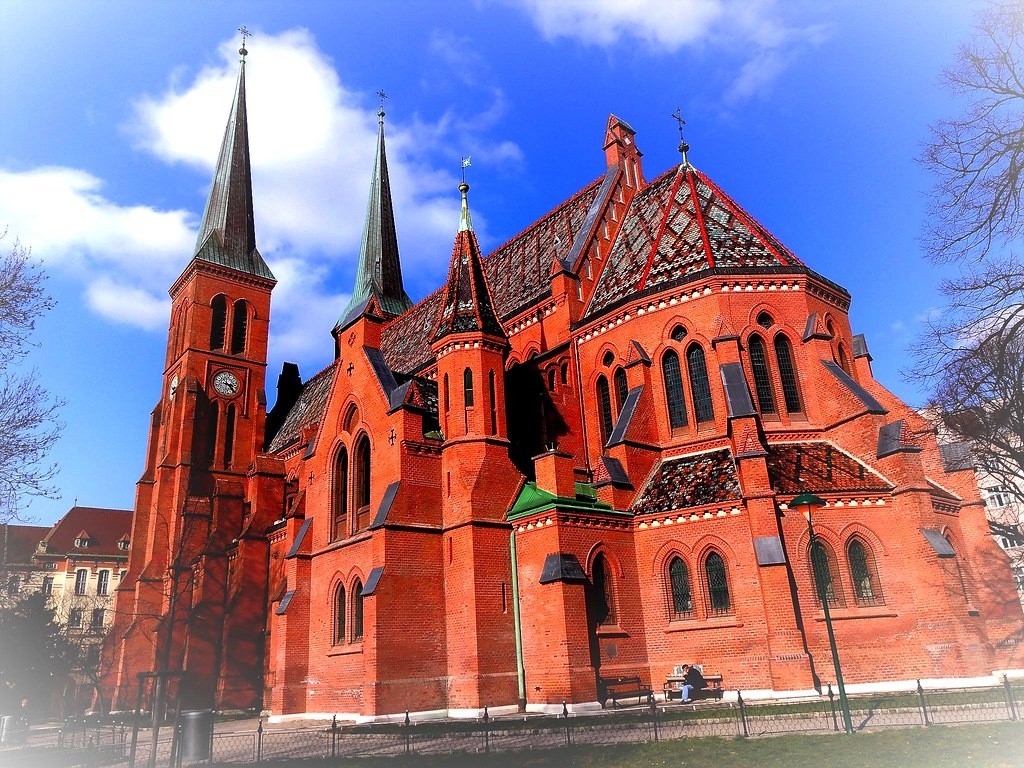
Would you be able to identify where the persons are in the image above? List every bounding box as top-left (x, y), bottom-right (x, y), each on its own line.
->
top-left (13, 699), bottom-right (31, 746)
top-left (678, 664), bottom-right (708, 705)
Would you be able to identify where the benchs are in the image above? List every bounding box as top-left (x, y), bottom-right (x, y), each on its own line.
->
top-left (599, 675), bottom-right (654, 710)
top-left (660, 674), bottom-right (724, 702)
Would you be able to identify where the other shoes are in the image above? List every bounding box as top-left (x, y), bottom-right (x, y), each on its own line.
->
top-left (678, 699), bottom-right (688, 705)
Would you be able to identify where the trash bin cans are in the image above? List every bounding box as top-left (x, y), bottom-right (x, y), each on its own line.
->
top-left (177, 707), bottom-right (211, 760)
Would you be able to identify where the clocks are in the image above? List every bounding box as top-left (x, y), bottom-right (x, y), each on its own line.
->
top-left (167, 372), bottom-right (179, 405)
top-left (211, 368), bottom-right (244, 399)
